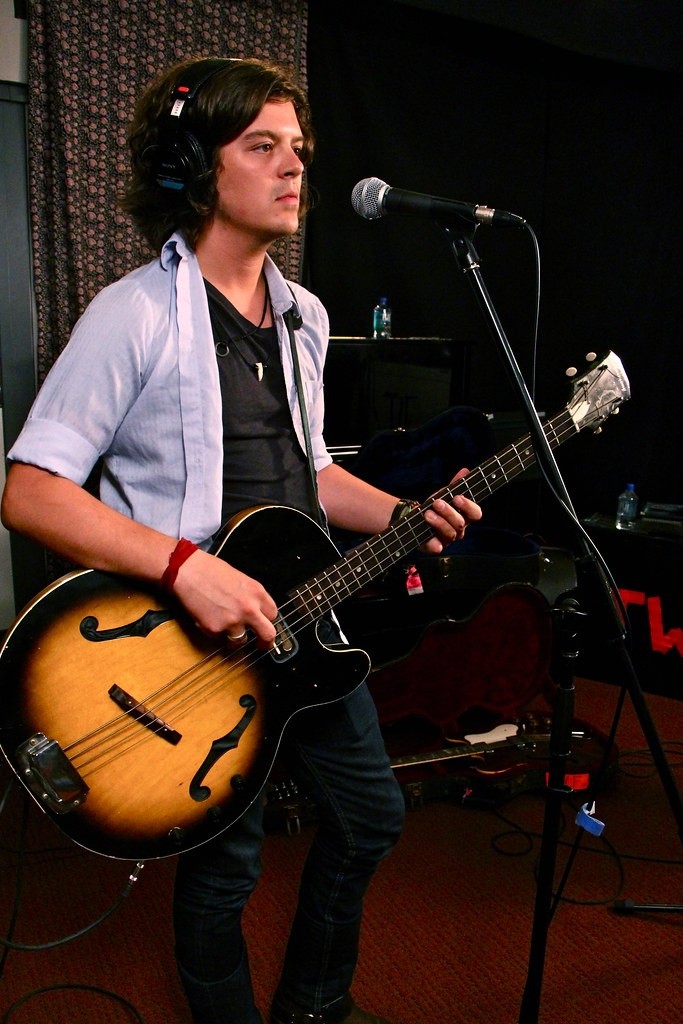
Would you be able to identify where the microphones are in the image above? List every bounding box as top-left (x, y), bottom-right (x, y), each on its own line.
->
top-left (351, 177), bottom-right (528, 230)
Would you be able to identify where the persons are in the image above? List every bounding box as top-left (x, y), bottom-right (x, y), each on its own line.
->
top-left (0, 59), bottom-right (482, 1024)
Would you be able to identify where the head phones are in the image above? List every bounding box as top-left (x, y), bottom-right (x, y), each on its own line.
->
top-left (144, 57), bottom-right (246, 199)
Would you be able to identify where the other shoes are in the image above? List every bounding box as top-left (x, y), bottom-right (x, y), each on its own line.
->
top-left (271, 1000), bottom-right (388, 1024)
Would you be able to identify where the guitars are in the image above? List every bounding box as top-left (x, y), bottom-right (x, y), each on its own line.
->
top-left (0, 340), bottom-right (634, 868)
top-left (266, 723), bottom-right (591, 809)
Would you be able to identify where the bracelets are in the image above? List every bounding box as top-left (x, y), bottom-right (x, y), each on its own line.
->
top-left (158, 537), bottom-right (200, 593)
top-left (388, 498), bottom-right (420, 527)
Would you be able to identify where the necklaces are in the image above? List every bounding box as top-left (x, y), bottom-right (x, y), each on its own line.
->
top-left (206, 275), bottom-right (277, 381)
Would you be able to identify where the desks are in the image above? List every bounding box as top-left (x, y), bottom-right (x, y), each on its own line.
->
top-left (555, 520), bottom-right (683, 701)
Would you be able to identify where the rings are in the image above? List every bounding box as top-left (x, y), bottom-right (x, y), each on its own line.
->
top-left (226, 629), bottom-right (247, 642)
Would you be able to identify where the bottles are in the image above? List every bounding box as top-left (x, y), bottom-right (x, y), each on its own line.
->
top-left (372, 297), bottom-right (393, 338)
top-left (615, 483), bottom-right (639, 530)
